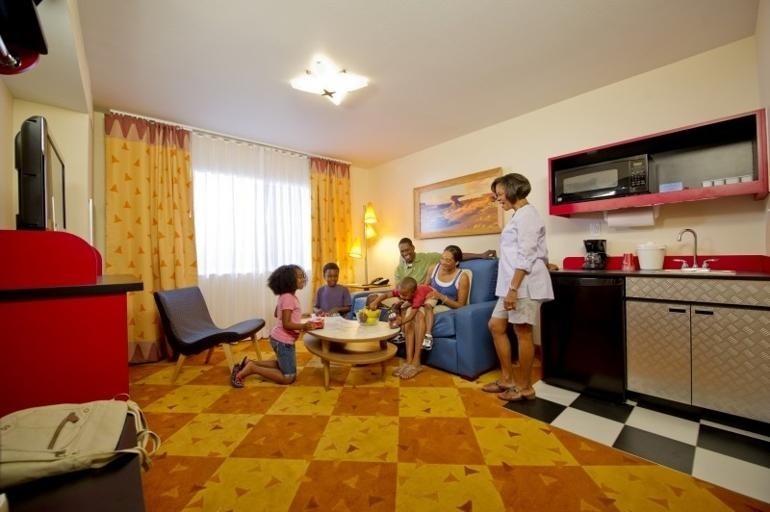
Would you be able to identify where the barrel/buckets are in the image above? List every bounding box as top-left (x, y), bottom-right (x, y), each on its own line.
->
top-left (635, 244), bottom-right (668, 271)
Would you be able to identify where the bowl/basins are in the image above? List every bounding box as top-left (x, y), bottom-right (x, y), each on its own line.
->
top-left (636, 242), bottom-right (666, 270)
top-left (356, 310), bottom-right (382, 326)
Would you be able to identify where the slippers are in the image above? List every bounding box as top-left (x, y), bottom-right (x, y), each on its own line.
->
top-left (239, 355), bottom-right (247, 370)
top-left (399, 365), bottom-right (423, 379)
top-left (391, 362), bottom-right (409, 377)
top-left (230, 362), bottom-right (245, 388)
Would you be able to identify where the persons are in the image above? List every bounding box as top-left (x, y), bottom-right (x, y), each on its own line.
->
top-left (313, 263), bottom-right (352, 317)
top-left (366, 239), bottom-right (497, 316)
top-left (391, 246), bottom-right (469, 379)
top-left (369, 277), bottom-right (437, 351)
top-left (482, 174), bottom-right (558, 401)
top-left (230, 265), bottom-right (311, 388)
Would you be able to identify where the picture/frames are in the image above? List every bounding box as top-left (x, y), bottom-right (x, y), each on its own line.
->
top-left (411, 167), bottom-right (506, 240)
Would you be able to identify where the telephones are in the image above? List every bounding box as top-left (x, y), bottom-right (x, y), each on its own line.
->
top-left (367, 277), bottom-right (389, 286)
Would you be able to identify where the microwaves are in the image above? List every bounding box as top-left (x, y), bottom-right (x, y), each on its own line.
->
top-left (555, 154), bottom-right (657, 207)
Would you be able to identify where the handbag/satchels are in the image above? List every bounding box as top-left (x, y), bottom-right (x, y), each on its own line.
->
top-left (0, 392), bottom-right (164, 491)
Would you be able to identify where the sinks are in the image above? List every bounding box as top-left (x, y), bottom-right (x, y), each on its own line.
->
top-left (664, 269), bottom-right (737, 277)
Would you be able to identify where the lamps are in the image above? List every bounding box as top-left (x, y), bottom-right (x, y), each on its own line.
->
top-left (286, 53), bottom-right (371, 108)
top-left (348, 201), bottom-right (382, 282)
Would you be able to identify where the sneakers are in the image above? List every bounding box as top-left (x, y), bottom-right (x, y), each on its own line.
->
top-left (497, 385), bottom-right (537, 401)
top-left (482, 379), bottom-right (518, 392)
top-left (392, 331), bottom-right (406, 344)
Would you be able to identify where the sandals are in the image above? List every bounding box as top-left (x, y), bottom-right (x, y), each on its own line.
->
top-left (421, 334), bottom-right (434, 350)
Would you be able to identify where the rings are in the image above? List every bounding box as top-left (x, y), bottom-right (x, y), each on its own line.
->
top-left (508, 307), bottom-right (512, 309)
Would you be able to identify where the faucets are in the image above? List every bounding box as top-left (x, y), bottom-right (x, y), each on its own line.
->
top-left (676, 227), bottom-right (698, 268)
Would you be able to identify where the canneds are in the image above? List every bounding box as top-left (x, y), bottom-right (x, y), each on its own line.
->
top-left (623, 252), bottom-right (633, 271)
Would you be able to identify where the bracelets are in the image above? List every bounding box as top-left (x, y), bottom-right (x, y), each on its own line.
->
top-left (441, 296), bottom-right (448, 304)
top-left (509, 285), bottom-right (519, 294)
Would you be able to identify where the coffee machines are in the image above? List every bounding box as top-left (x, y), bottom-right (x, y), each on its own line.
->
top-left (583, 240), bottom-right (607, 270)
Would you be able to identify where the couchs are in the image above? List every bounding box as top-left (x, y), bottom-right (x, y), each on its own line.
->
top-left (345, 251), bottom-right (522, 382)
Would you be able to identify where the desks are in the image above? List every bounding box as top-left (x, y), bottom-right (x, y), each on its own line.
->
top-left (301, 313), bottom-right (404, 389)
top-left (0, 226), bottom-right (145, 420)
top-left (0, 402), bottom-right (147, 510)
top-left (338, 280), bottom-right (393, 291)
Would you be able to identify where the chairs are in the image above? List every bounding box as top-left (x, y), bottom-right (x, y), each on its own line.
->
top-left (151, 285), bottom-right (266, 387)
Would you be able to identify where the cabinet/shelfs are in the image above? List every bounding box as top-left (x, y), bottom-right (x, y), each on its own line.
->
top-left (542, 107), bottom-right (769, 222)
top-left (625, 274), bottom-right (770, 439)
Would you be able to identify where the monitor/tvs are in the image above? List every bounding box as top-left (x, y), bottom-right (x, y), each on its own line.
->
top-left (14, 115), bottom-right (66, 232)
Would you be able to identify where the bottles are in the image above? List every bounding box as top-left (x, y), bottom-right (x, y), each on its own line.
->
top-left (623, 253), bottom-right (635, 272)
